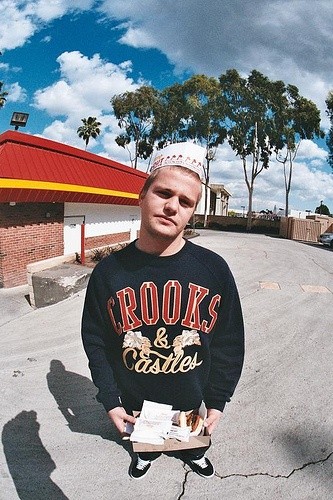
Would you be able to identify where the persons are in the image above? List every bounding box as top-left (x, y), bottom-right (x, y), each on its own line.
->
top-left (82, 143), bottom-right (244, 479)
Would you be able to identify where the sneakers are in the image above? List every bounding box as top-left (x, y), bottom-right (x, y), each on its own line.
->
top-left (189, 455), bottom-right (215, 479)
top-left (129, 452), bottom-right (164, 479)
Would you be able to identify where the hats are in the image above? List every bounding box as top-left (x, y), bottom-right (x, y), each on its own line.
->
top-left (150, 142), bottom-right (208, 182)
